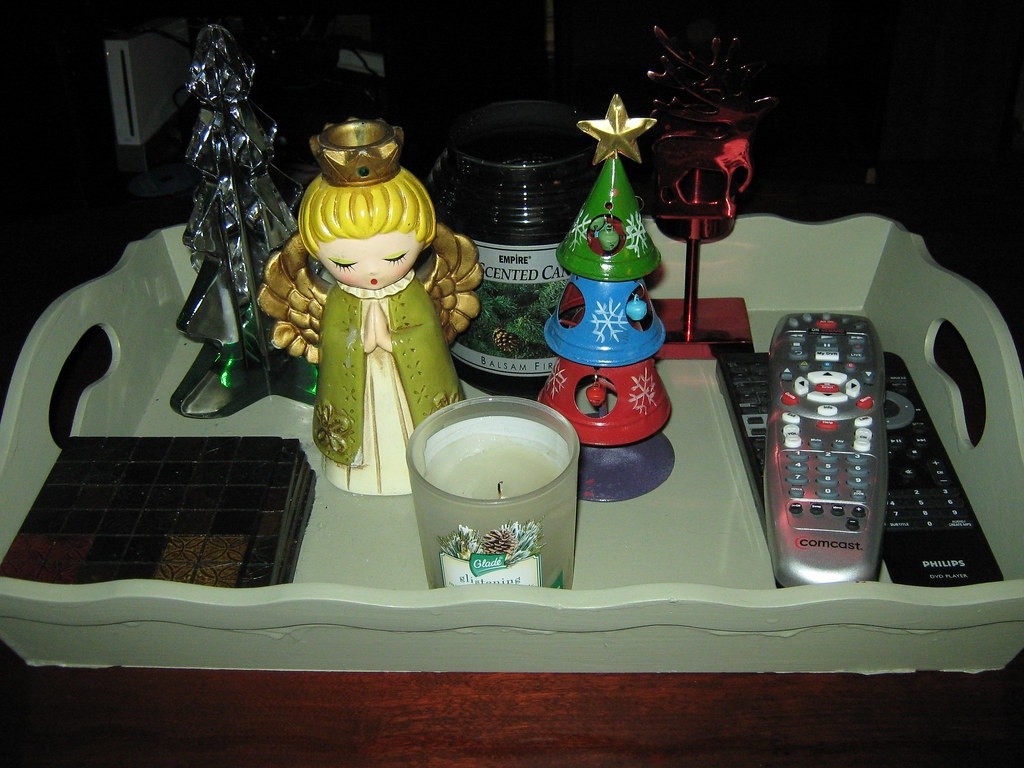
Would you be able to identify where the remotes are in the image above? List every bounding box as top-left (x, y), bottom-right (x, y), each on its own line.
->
top-left (722, 311), bottom-right (1005, 588)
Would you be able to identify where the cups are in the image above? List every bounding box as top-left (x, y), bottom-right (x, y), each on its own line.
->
top-left (406, 397), bottom-right (579, 591)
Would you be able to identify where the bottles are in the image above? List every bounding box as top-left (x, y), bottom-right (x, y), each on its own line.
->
top-left (426, 98), bottom-right (604, 406)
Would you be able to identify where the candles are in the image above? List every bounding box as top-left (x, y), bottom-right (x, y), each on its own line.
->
top-left (406, 395), bottom-right (582, 591)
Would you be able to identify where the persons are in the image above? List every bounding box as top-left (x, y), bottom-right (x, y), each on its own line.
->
top-left (257, 116), bottom-right (484, 494)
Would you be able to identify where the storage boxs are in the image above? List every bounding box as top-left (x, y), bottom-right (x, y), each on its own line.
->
top-left (0, 212), bottom-right (1024, 674)
